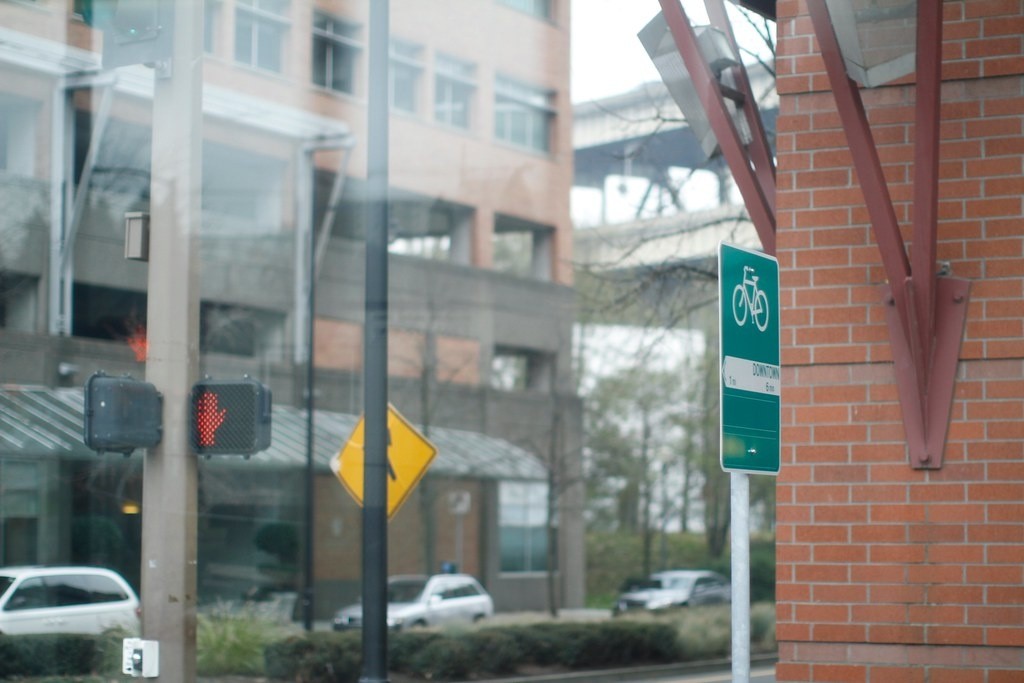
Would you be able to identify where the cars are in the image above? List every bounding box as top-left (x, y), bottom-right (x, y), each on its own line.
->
top-left (0, 566), bottom-right (142, 637)
top-left (611, 570), bottom-right (732, 617)
top-left (331, 573), bottom-right (495, 630)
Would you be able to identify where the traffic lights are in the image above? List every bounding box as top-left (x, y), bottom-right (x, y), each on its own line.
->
top-left (191, 379), bottom-right (271, 455)
